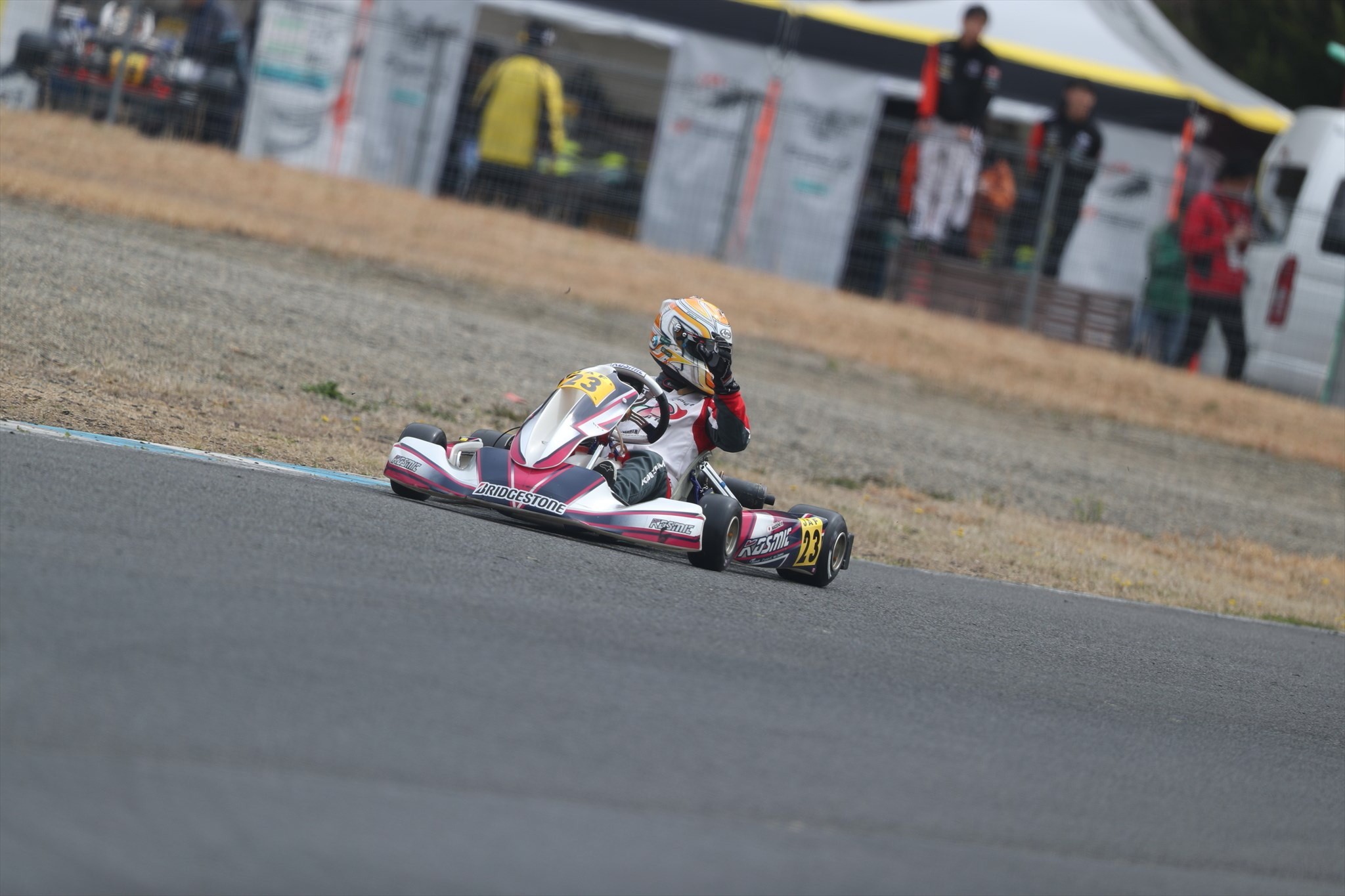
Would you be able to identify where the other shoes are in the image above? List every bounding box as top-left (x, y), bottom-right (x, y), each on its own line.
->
top-left (591, 459), bottom-right (617, 488)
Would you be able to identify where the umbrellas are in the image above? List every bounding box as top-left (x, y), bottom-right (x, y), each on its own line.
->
top-left (736, 0), bottom-right (1296, 135)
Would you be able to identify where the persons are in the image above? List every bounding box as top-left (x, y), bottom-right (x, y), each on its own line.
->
top-left (1132, 194), bottom-right (1192, 366)
top-left (969, 157), bottom-right (1013, 260)
top-left (99, 0), bottom-right (154, 43)
top-left (1003, 78), bottom-right (1102, 279)
top-left (467, 20), bottom-right (571, 209)
top-left (182, 0), bottom-right (251, 143)
top-left (579, 296), bottom-right (751, 506)
top-left (1179, 161), bottom-right (1254, 382)
top-left (911, 5), bottom-right (1001, 260)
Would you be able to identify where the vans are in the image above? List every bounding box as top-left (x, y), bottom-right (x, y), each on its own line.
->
top-left (1208, 105), bottom-right (1345, 406)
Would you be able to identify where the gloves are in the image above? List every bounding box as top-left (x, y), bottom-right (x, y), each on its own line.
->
top-left (697, 331), bottom-right (736, 388)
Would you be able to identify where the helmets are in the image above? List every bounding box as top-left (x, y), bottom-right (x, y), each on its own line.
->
top-left (649, 295), bottom-right (733, 397)
